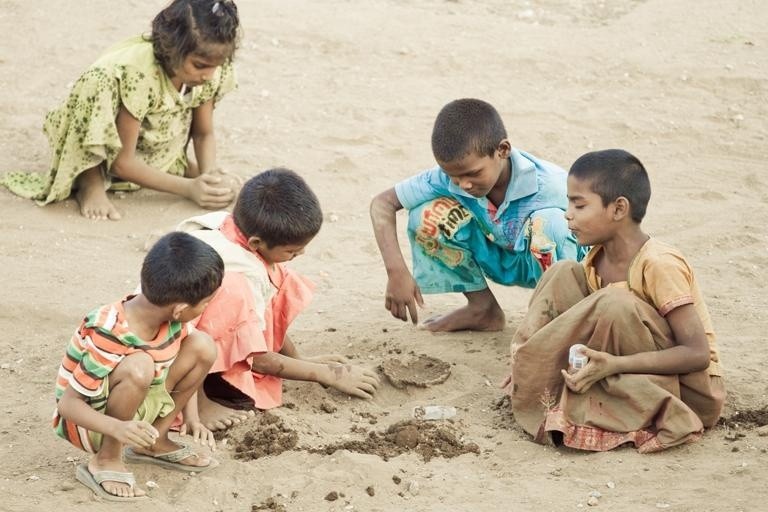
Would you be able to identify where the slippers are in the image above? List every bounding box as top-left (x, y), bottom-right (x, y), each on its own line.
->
top-left (75, 463), bottom-right (148, 502)
top-left (124, 441), bottom-right (219, 473)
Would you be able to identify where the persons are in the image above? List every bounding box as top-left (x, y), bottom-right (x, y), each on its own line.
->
top-left (49, 230), bottom-right (227, 504)
top-left (366, 97), bottom-right (594, 334)
top-left (0, 0), bottom-right (246, 222)
top-left (133, 164), bottom-right (382, 433)
top-left (497, 147), bottom-right (727, 456)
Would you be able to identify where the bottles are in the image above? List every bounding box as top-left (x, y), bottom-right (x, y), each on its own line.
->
top-left (566, 343), bottom-right (589, 376)
top-left (413, 406), bottom-right (456, 421)
